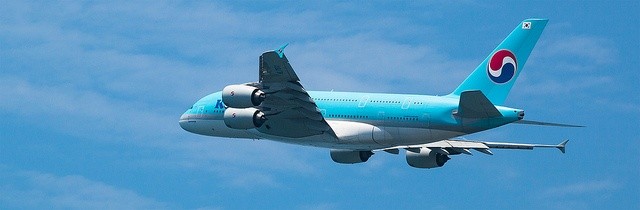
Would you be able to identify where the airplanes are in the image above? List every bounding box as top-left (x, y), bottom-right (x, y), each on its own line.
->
top-left (178, 18), bottom-right (589, 169)
